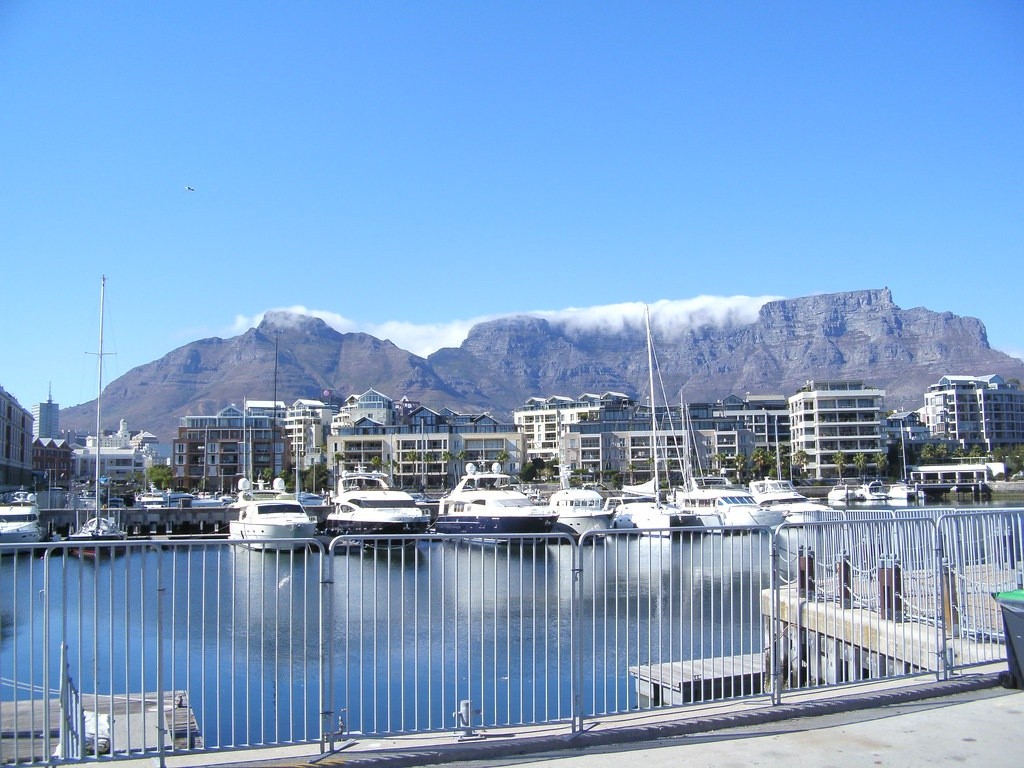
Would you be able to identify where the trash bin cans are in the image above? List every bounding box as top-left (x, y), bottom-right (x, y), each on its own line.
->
top-left (990, 589), bottom-right (1024, 691)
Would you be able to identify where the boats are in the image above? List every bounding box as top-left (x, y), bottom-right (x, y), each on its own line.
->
top-left (828, 483), bottom-right (857, 501)
top-left (886, 483), bottom-right (916, 503)
top-left (855, 481), bottom-right (890, 500)
top-left (0, 494), bottom-right (47, 553)
top-left (134, 304), bottom-right (834, 552)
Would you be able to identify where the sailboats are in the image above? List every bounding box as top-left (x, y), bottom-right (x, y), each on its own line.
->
top-left (68, 273), bottom-right (130, 557)
